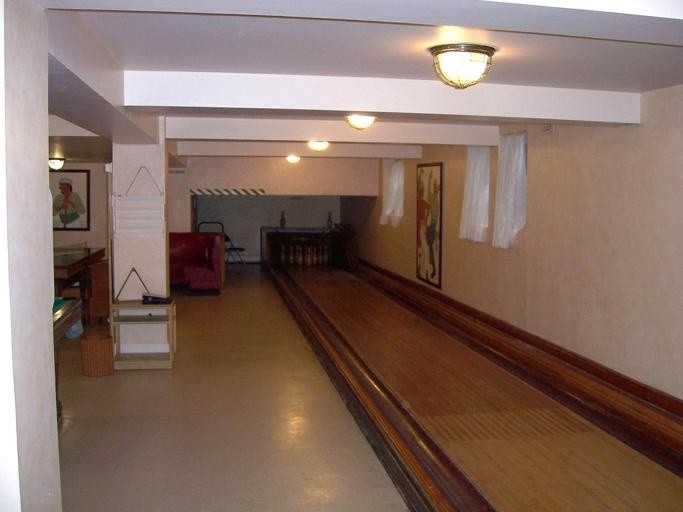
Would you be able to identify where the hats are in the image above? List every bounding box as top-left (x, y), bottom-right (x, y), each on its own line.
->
top-left (59, 178), bottom-right (72, 185)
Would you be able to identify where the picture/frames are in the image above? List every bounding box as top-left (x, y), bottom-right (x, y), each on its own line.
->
top-left (417, 162), bottom-right (443, 288)
top-left (49, 170), bottom-right (91, 231)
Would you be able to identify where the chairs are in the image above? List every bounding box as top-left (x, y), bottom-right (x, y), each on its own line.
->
top-left (224, 233), bottom-right (246, 266)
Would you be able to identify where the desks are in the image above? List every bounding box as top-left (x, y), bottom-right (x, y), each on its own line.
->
top-left (54, 247), bottom-right (105, 333)
top-left (53, 297), bottom-right (83, 434)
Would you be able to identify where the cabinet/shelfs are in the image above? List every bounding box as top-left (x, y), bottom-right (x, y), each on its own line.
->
top-left (109, 299), bottom-right (177, 368)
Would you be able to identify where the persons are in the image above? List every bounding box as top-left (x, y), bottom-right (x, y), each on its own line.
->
top-left (53, 177), bottom-right (85, 228)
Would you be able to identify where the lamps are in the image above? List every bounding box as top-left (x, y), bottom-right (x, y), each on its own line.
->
top-left (48, 158), bottom-right (65, 171)
top-left (306, 141), bottom-right (332, 153)
top-left (343, 114), bottom-right (377, 131)
top-left (428, 43), bottom-right (496, 90)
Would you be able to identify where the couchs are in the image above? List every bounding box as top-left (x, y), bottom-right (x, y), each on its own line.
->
top-left (169, 233), bottom-right (225, 294)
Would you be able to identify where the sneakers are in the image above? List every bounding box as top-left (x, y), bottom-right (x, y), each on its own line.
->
top-left (431, 272), bottom-right (436, 278)
